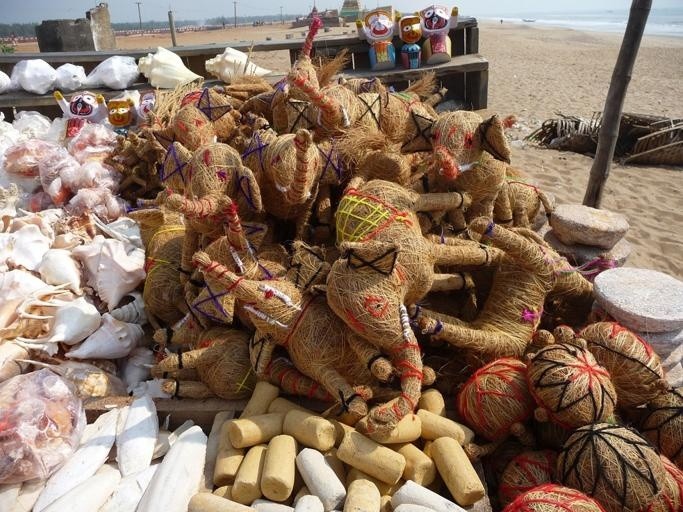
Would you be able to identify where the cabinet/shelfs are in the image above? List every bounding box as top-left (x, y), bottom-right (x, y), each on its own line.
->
top-left (0, 15), bottom-right (488, 124)
